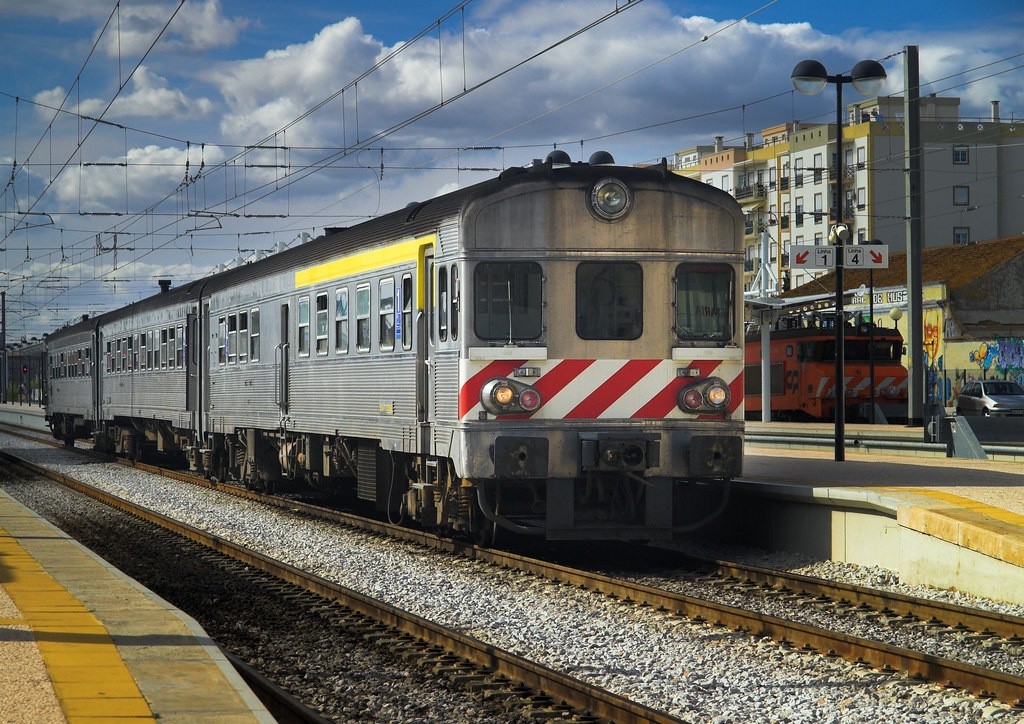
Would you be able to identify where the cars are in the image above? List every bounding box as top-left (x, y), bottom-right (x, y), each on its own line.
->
top-left (955, 379), bottom-right (1024, 417)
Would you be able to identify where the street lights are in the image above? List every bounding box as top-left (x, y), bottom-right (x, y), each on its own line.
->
top-left (790, 59), bottom-right (888, 463)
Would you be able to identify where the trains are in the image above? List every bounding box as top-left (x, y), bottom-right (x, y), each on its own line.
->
top-left (746, 308), bottom-right (908, 425)
top-left (42, 149), bottom-right (746, 550)
